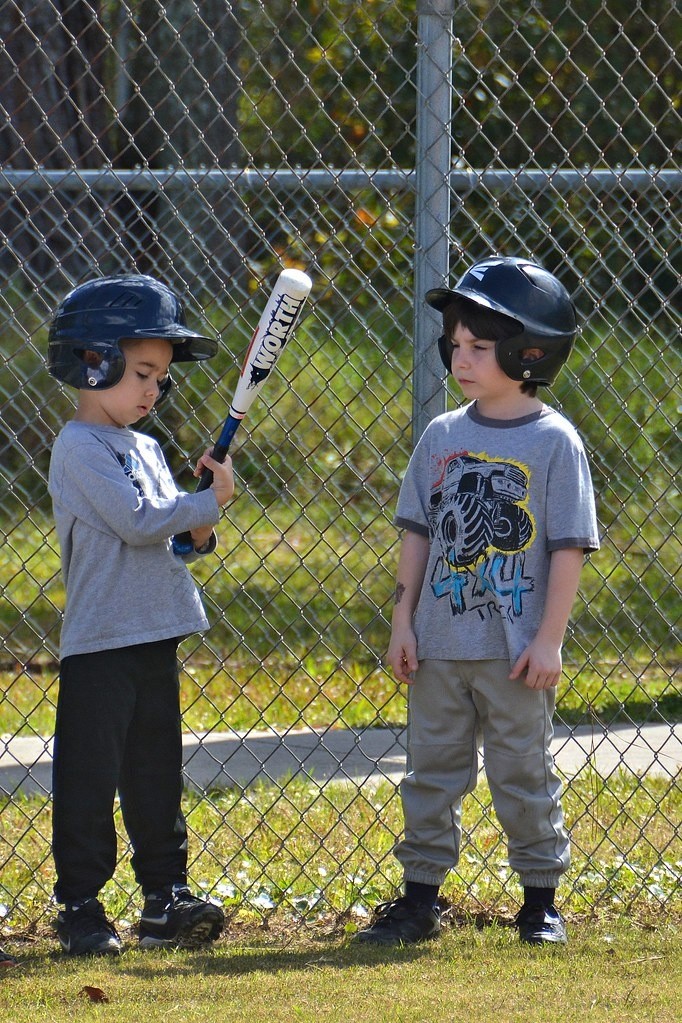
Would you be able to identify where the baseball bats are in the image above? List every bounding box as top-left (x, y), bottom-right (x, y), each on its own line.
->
top-left (169, 266), bottom-right (315, 558)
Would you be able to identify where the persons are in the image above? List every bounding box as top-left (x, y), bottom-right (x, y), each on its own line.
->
top-left (46, 273), bottom-right (237, 956)
top-left (350, 258), bottom-right (600, 946)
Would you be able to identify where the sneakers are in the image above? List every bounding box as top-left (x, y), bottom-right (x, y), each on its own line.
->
top-left (139, 884), bottom-right (225, 950)
top-left (514, 904), bottom-right (566, 946)
top-left (352, 896), bottom-right (442, 949)
top-left (53, 898), bottom-right (122, 958)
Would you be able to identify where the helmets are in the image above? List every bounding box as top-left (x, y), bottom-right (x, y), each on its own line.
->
top-left (47, 274), bottom-right (218, 411)
top-left (425, 256), bottom-right (578, 387)
